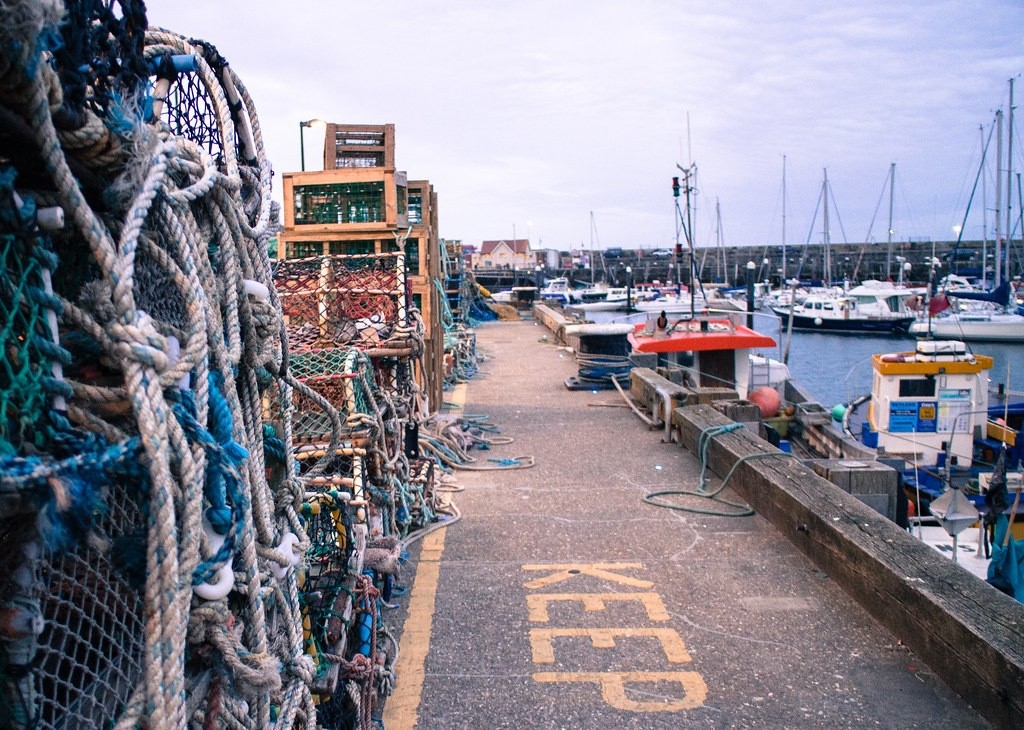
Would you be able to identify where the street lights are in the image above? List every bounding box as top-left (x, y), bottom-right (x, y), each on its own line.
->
top-left (298, 117), bottom-right (327, 169)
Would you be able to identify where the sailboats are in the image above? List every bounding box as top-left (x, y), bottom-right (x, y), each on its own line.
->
top-left (490, 110), bottom-right (1024, 343)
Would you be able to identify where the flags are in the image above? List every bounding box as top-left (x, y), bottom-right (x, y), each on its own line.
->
top-left (929, 291), bottom-right (949, 317)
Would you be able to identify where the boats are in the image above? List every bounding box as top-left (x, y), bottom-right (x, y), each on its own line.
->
top-left (830, 243), bottom-right (1024, 520)
top-left (900, 410), bottom-right (1023, 603)
